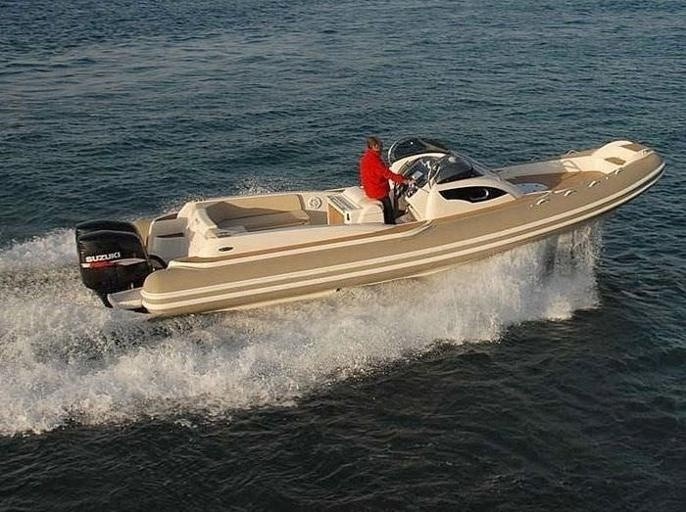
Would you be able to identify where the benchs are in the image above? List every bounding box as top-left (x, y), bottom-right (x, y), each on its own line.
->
top-left (205, 195), bottom-right (312, 234)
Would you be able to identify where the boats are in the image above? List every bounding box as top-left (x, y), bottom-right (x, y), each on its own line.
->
top-left (75, 136), bottom-right (667, 317)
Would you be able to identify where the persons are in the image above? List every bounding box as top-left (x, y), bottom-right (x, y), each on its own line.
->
top-left (359, 136), bottom-right (417, 224)
